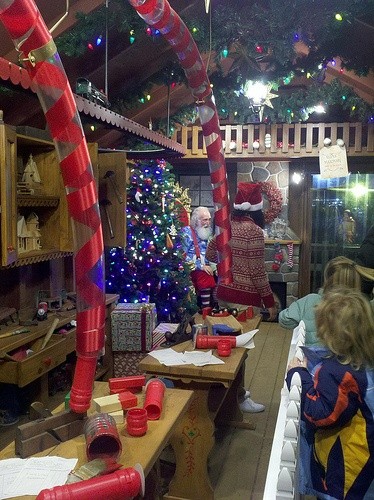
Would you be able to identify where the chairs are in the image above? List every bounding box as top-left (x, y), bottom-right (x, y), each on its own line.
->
top-left (110, 301), bottom-right (179, 377)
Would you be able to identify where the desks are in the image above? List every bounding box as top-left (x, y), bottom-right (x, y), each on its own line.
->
top-left (137, 314), bottom-right (264, 500)
top-left (0, 379), bottom-right (196, 500)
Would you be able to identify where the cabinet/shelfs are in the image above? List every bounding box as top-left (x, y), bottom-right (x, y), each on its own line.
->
top-left (0, 124), bottom-right (126, 406)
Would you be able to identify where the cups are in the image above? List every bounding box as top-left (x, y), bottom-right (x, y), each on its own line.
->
top-left (217, 339), bottom-right (232, 356)
top-left (126, 407), bottom-right (148, 436)
top-left (192, 324), bottom-right (208, 340)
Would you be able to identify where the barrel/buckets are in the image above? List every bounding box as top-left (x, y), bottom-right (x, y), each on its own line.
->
top-left (83, 412), bottom-right (123, 465)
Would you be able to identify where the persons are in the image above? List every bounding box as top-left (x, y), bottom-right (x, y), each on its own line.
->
top-left (342, 210), bottom-right (356, 244)
top-left (285, 285), bottom-right (374, 500)
top-left (181, 206), bottom-right (218, 318)
top-left (279, 257), bottom-right (374, 354)
top-left (205, 182), bottom-right (276, 414)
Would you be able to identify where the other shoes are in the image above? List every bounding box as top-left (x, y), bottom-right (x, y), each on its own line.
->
top-left (240, 398), bottom-right (265, 412)
top-left (245, 391), bottom-right (250, 396)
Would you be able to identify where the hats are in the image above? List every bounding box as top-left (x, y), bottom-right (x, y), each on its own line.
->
top-left (234, 182), bottom-right (263, 211)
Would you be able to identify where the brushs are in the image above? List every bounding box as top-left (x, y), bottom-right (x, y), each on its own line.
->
top-left (0, 325), bottom-right (31, 339)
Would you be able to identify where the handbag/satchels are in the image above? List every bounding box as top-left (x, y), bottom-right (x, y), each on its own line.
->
top-left (190, 269), bottom-right (216, 290)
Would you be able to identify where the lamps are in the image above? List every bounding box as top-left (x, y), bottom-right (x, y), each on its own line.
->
top-left (246, 82), bottom-right (267, 117)
